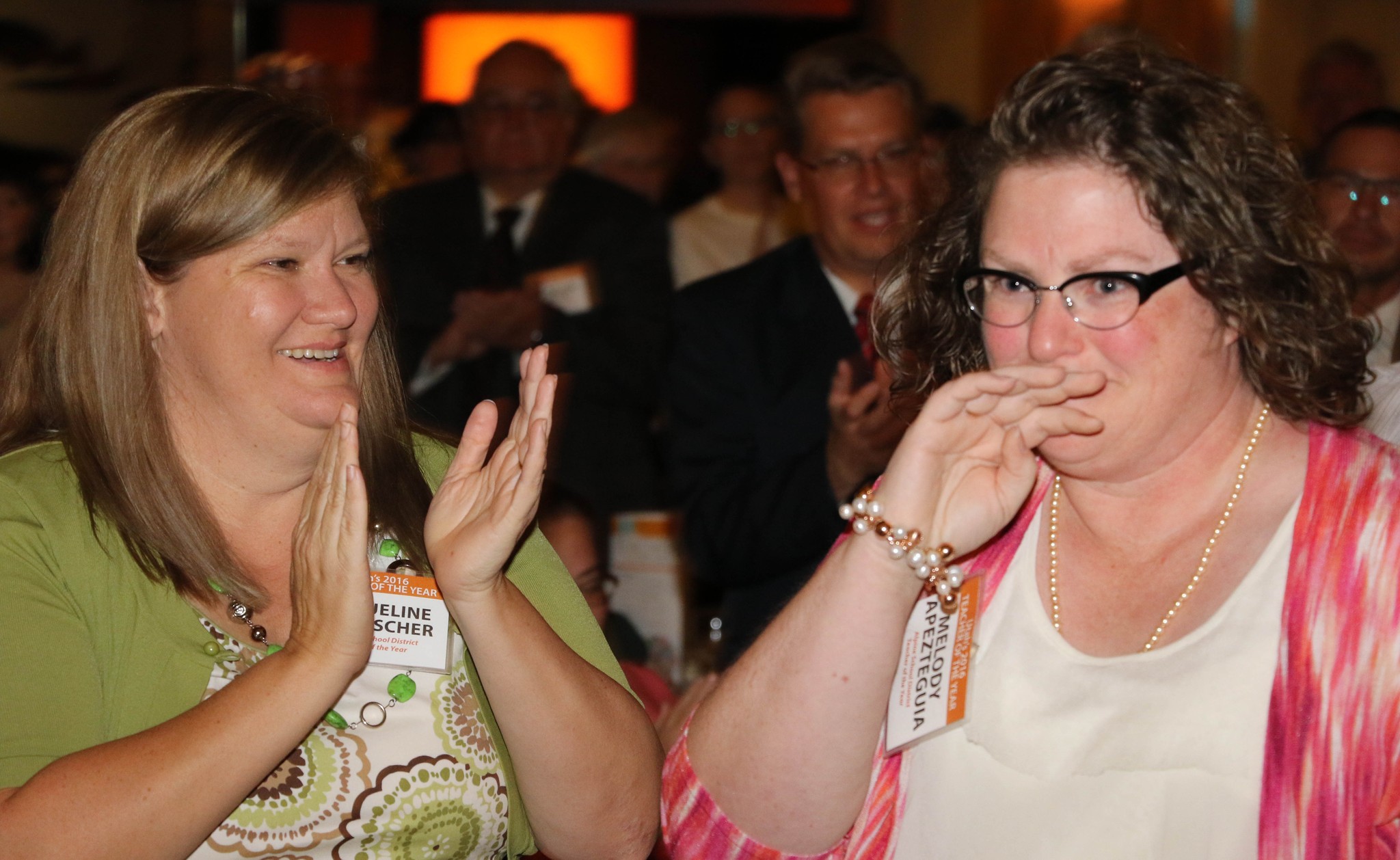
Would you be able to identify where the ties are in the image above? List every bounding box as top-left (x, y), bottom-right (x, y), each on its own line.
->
top-left (485, 206), bottom-right (521, 265)
top-left (854, 293), bottom-right (883, 370)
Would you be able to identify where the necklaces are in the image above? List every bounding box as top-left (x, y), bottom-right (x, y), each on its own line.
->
top-left (1050, 395), bottom-right (1277, 656)
top-left (205, 503), bottom-right (451, 729)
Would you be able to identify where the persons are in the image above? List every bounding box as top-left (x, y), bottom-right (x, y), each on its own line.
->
top-left (0, 87), bottom-right (663, 860)
top-left (657, 28), bottom-right (1400, 860)
top-left (1, 32), bottom-right (1398, 727)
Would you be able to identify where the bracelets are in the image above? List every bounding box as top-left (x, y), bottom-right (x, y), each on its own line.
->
top-left (818, 483), bottom-right (983, 623)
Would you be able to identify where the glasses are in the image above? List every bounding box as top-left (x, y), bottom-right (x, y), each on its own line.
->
top-left (802, 142), bottom-right (924, 183)
top-left (1319, 169), bottom-right (1400, 210)
top-left (961, 255), bottom-right (1211, 330)
